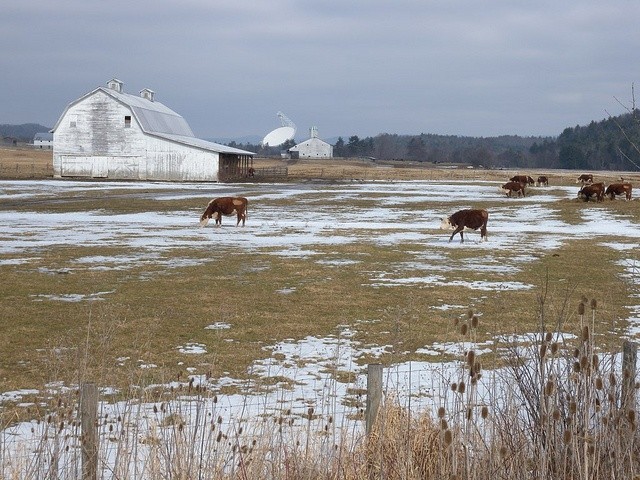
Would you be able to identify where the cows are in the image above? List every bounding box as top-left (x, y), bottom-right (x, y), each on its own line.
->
top-left (439, 209), bottom-right (488, 243)
top-left (575, 175), bottom-right (593, 190)
top-left (200, 198), bottom-right (247, 227)
top-left (577, 187), bottom-right (601, 205)
top-left (537, 176), bottom-right (548, 187)
top-left (501, 182), bottom-right (525, 198)
top-left (604, 184), bottom-right (632, 202)
top-left (591, 182), bottom-right (605, 198)
top-left (510, 176), bottom-right (535, 187)
